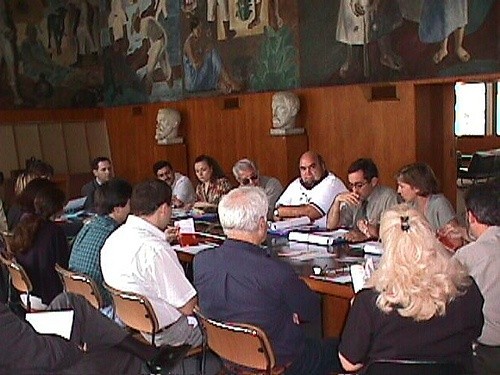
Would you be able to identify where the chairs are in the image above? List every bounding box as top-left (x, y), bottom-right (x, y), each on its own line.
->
top-left (0, 231), bottom-right (481, 375)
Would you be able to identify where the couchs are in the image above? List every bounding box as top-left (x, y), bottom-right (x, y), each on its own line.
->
top-left (455, 149), bottom-right (500, 190)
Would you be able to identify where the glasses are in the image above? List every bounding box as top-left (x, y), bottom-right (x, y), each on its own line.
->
top-left (347, 179), bottom-right (371, 189)
top-left (242, 173), bottom-right (258, 185)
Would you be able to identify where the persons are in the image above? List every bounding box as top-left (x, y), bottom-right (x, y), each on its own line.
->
top-left (275, 149), bottom-right (351, 229)
top-left (1, 153), bottom-right (231, 374)
top-left (194, 186), bottom-right (338, 374)
top-left (327, 158), bottom-right (398, 242)
top-left (359, 162), bottom-right (457, 242)
top-left (153, 107), bottom-right (184, 144)
top-left (233, 157), bottom-right (285, 223)
top-left (446, 186), bottom-right (500, 372)
top-left (338, 203), bottom-right (481, 375)
top-left (270, 91), bottom-right (301, 131)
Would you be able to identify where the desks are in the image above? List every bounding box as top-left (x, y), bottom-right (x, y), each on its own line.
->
top-left (461, 155), bottom-right (473, 161)
top-left (51, 208), bottom-right (385, 340)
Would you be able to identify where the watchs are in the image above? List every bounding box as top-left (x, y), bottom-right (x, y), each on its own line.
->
top-left (274, 205), bottom-right (280, 220)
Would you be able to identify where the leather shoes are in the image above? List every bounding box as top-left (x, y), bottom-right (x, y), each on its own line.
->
top-left (146, 343), bottom-right (192, 374)
